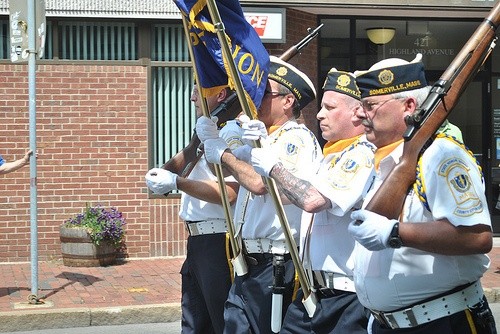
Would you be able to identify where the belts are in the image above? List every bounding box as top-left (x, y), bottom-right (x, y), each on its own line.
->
top-left (243, 239), bottom-right (300, 255)
top-left (305, 269), bottom-right (357, 293)
top-left (370, 279), bottom-right (484, 330)
top-left (184, 219), bottom-right (228, 236)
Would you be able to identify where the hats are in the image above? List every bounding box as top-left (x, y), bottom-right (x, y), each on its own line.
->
top-left (353, 53), bottom-right (427, 99)
top-left (322, 67), bottom-right (361, 100)
top-left (267, 54), bottom-right (316, 110)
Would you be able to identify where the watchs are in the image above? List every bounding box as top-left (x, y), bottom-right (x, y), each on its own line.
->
top-left (389, 222), bottom-right (403, 249)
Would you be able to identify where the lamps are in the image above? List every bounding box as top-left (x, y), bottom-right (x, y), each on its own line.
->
top-left (366, 27), bottom-right (396, 45)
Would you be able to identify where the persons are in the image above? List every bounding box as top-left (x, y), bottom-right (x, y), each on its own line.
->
top-left (237, 68), bottom-right (369, 334)
top-left (196, 56), bottom-right (323, 334)
top-left (352, 51), bottom-right (497, 334)
top-left (146, 78), bottom-right (242, 334)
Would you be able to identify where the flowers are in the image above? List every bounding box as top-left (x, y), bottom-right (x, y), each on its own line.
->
top-left (61, 201), bottom-right (128, 257)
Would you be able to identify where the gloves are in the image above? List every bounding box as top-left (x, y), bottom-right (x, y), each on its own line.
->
top-left (348, 210), bottom-right (398, 252)
top-left (196, 116), bottom-right (219, 143)
top-left (204, 137), bottom-right (232, 166)
top-left (250, 147), bottom-right (282, 178)
top-left (239, 114), bottom-right (269, 149)
top-left (144, 167), bottom-right (178, 195)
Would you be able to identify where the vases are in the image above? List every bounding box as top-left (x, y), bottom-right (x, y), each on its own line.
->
top-left (61, 228), bottom-right (117, 267)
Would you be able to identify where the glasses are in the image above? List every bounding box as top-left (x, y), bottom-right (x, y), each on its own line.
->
top-left (359, 96), bottom-right (401, 109)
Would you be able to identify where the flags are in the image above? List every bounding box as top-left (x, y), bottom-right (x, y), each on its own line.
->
top-left (206, 0), bottom-right (269, 122)
top-left (173, 0), bottom-right (229, 95)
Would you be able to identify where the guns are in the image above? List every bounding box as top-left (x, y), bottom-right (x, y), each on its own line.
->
top-left (352, 1), bottom-right (500, 226)
top-left (151, 22), bottom-right (325, 196)
